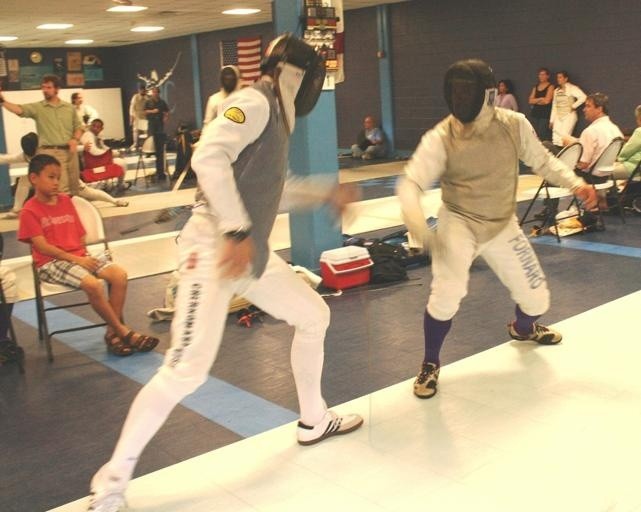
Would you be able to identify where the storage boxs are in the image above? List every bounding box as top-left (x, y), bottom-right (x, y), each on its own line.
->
top-left (320, 244), bottom-right (374, 291)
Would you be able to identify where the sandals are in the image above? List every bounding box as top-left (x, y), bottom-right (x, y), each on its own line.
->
top-left (105, 330), bottom-right (158, 355)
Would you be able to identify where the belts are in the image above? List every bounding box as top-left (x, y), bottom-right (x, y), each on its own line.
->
top-left (40, 145), bottom-right (70, 150)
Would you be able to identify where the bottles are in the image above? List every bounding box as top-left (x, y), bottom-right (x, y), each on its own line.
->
top-left (91, 250), bottom-right (113, 265)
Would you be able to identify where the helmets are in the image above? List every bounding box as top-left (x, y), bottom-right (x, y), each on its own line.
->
top-left (444, 59), bottom-right (498, 140)
top-left (220, 64), bottom-right (240, 98)
top-left (260, 33), bottom-right (326, 135)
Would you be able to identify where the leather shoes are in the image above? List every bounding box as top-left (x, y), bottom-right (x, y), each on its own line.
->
top-left (535, 209), bottom-right (557, 219)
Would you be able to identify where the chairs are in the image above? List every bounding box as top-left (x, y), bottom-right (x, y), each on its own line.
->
top-left (616, 160), bottom-right (640, 222)
top-left (81, 147), bottom-right (123, 193)
top-left (31, 196), bottom-right (125, 363)
top-left (518, 144), bottom-right (586, 243)
top-left (1, 280), bottom-right (26, 377)
top-left (565, 137), bottom-right (625, 230)
top-left (134, 137), bottom-right (166, 189)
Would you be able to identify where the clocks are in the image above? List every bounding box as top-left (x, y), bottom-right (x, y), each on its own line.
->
top-left (29, 50), bottom-right (42, 64)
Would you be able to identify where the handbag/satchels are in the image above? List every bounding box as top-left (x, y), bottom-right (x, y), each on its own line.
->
top-left (366, 239), bottom-right (410, 284)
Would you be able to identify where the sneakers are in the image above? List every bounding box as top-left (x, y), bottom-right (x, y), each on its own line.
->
top-left (297, 411), bottom-right (363, 446)
top-left (508, 320), bottom-right (562, 345)
top-left (414, 362), bottom-right (440, 398)
top-left (89, 466), bottom-right (128, 512)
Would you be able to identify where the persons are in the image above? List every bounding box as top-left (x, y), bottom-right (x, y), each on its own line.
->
top-left (203, 65), bottom-right (245, 132)
top-left (495, 69), bottom-right (640, 224)
top-left (353, 116), bottom-right (382, 159)
top-left (130, 85), bottom-right (169, 149)
top-left (396, 59), bottom-right (601, 399)
top-left (16, 154), bottom-right (160, 355)
top-left (90, 34), bottom-right (367, 512)
top-left (1, 77), bottom-right (129, 209)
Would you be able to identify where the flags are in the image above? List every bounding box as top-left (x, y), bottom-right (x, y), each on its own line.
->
top-left (220, 39), bottom-right (262, 89)
